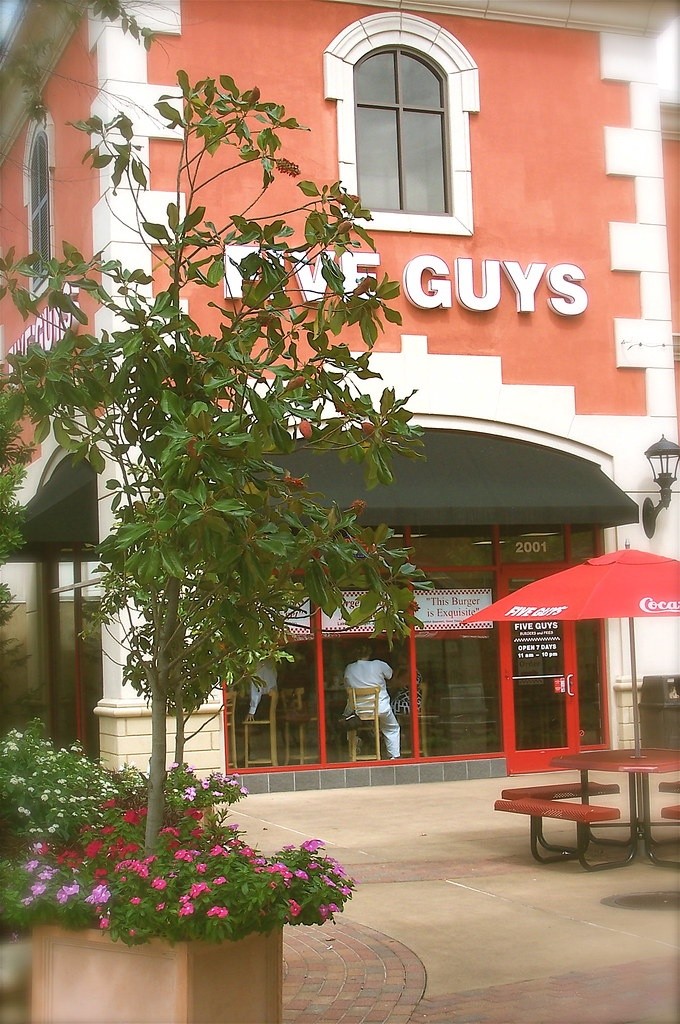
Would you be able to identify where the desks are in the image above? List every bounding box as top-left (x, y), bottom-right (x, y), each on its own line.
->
top-left (552, 747), bottom-right (680, 871)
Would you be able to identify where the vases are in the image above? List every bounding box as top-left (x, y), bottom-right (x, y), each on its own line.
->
top-left (196, 807), bottom-right (215, 829)
top-left (30, 921), bottom-right (284, 1024)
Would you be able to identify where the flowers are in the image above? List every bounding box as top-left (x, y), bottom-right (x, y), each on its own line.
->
top-left (0, 713), bottom-right (358, 945)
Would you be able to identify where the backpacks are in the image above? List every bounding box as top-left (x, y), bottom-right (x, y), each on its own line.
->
top-left (281, 659), bottom-right (303, 689)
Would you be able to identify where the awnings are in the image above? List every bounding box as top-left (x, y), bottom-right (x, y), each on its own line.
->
top-left (263, 427), bottom-right (638, 531)
top-left (3, 454), bottom-right (99, 563)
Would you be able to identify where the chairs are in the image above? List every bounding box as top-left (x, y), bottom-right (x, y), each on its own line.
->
top-left (227, 683), bottom-right (429, 767)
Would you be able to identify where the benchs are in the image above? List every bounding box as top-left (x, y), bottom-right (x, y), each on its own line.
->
top-left (492, 781), bottom-right (680, 872)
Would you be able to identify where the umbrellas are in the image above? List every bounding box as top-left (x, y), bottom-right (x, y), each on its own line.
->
top-left (459, 538), bottom-right (680, 841)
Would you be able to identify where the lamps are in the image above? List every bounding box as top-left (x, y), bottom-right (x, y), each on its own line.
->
top-left (642, 433), bottom-right (680, 540)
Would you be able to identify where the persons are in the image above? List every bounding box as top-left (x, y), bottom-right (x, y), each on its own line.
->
top-left (245, 641), bottom-right (424, 758)
